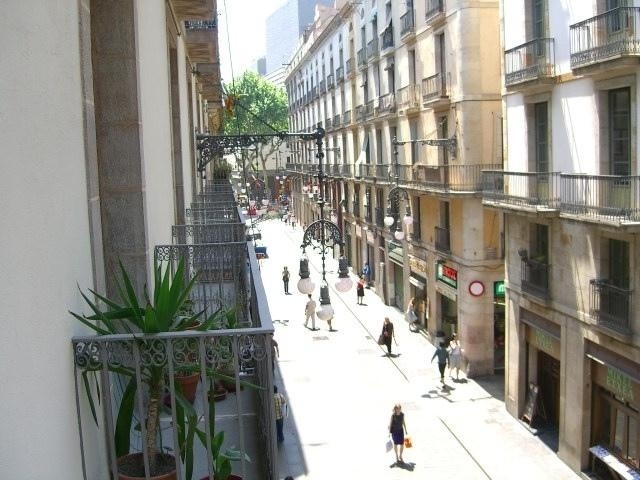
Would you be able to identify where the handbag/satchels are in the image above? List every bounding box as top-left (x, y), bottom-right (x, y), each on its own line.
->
top-left (404, 438), bottom-right (411, 448)
top-left (386, 440), bottom-right (393, 451)
top-left (378, 336), bottom-right (385, 344)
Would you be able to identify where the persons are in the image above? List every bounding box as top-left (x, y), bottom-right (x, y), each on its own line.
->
top-left (272, 385), bottom-right (289, 442)
top-left (303, 292), bottom-right (317, 331)
top-left (232, 189), bottom-right (308, 233)
top-left (447, 333), bottom-right (465, 380)
top-left (405, 297), bottom-right (418, 331)
top-left (361, 262), bottom-right (370, 289)
top-left (356, 277), bottom-right (365, 305)
top-left (430, 339), bottom-right (451, 390)
top-left (381, 316), bottom-right (394, 356)
top-left (282, 265), bottom-right (291, 295)
top-left (388, 404), bottom-right (408, 464)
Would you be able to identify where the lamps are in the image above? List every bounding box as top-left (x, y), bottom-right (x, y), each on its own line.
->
top-left (331, 228), bottom-right (350, 293)
top-left (383, 103), bottom-right (458, 240)
top-left (290, 222), bottom-right (317, 295)
top-left (311, 219), bottom-right (337, 318)
